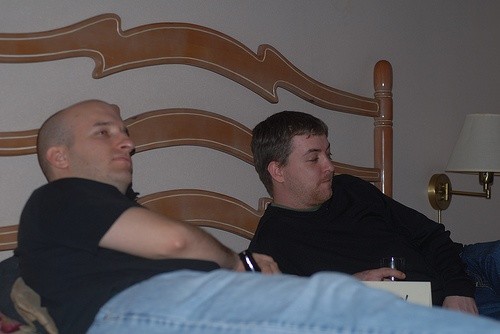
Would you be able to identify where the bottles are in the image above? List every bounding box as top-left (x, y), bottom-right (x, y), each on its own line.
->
top-left (238, 249), bottom-right (263, 273)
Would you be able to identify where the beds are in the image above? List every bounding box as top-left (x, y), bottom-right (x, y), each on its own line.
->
top-left (0, 12), bottom-right (393, 334)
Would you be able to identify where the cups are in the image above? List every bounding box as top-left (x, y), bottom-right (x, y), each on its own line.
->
top-left (381, 256), bottom-right (406, 282)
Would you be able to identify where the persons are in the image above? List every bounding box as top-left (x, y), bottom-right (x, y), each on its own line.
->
top-left (18, 100), bottom-right (500, 334)
top-left (247, 110), bottom-right (500, 321)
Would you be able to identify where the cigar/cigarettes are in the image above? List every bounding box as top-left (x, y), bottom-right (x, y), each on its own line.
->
top-left (390, 257), bottom-right (396, 282)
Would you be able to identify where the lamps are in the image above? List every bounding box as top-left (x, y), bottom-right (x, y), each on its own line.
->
top-left (427, 113), bottom-right (500, 210)
